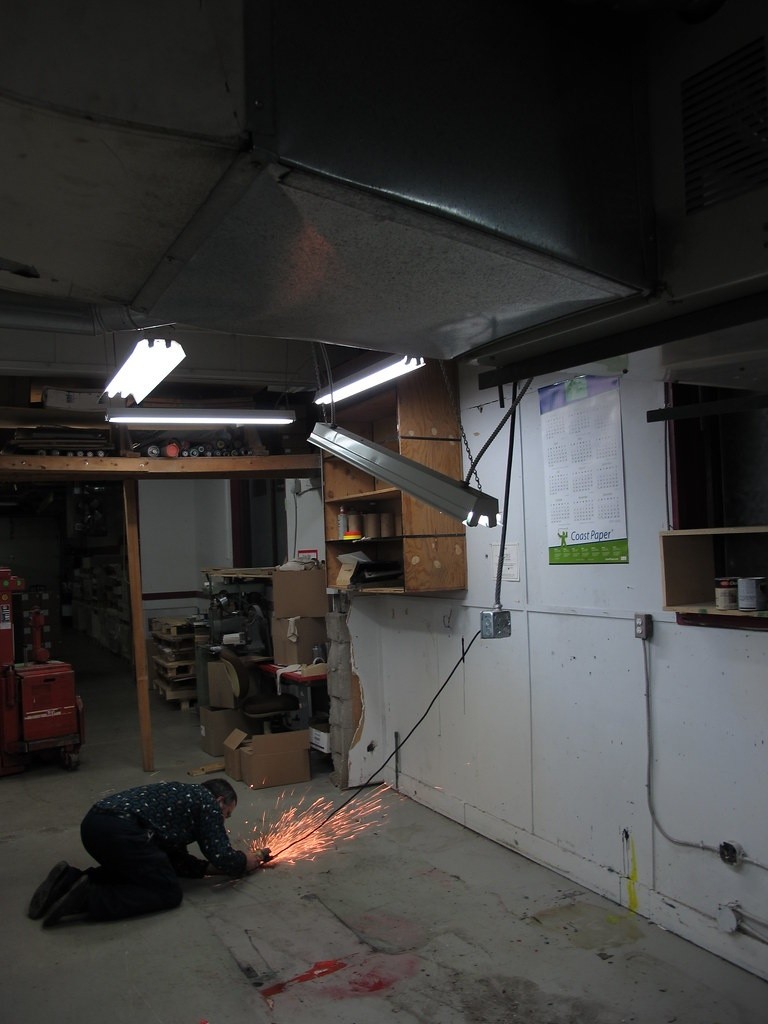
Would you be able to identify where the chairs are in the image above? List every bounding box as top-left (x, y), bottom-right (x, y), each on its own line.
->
top-left (218, 651), bottom-right (301, 734)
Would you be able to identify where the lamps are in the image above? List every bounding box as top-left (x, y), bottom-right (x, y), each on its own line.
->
top-left (95, 337), bottom-right (188, 405)
top-left (313, 354), bottom-right (427, 404)
top-left (305, 422), bottom-right (505, 531)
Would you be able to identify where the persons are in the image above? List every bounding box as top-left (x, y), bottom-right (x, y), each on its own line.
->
top-left (28, 777), bottom-right (263, 931)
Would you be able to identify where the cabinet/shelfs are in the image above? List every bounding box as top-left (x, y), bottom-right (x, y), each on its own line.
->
top-left (323, 359), bottom-right (468, 593)
top-left (658, 530), bottom-right (767, 620)
top-left (0, 373), bottom-right (321, 480)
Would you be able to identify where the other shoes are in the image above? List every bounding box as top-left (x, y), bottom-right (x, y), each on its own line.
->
top-left (42, 872), bottom-right (94, 927)
top-left (29, 862), bottom-right (83, 920)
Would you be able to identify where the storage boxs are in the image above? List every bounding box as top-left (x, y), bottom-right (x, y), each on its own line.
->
top-left (223, 731), bottom-right (254, 782)
top-left (240, 729), bottom-right (314, 789)
